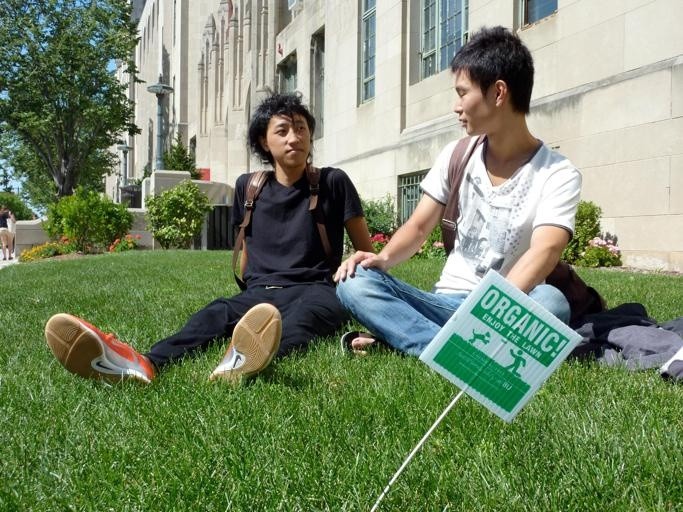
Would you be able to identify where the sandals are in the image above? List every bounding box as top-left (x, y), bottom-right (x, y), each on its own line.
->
top-left (342, 330), bottom-right (383, 356)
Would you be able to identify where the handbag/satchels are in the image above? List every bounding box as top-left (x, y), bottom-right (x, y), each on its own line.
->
top-left (546, 263), bottom-right (605, 314)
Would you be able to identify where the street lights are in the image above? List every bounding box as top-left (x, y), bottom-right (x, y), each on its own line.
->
top-left (146, 74), bottom-right (174, 171)
top-left (117, 141), bottom-right (134, 187)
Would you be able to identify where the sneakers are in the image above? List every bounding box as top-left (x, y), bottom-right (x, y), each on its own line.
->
top-left (207, 304), bottom-right (281, 390)
top-left (45, 312), bottom-right (155, 386)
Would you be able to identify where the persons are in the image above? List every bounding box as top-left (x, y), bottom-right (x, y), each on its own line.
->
top-left (0, 207), bottom-right (16, 260)
top-left (333, 26), bottom-right (583, 360)
top-left (45, 85), bottom-right (376, 389)
